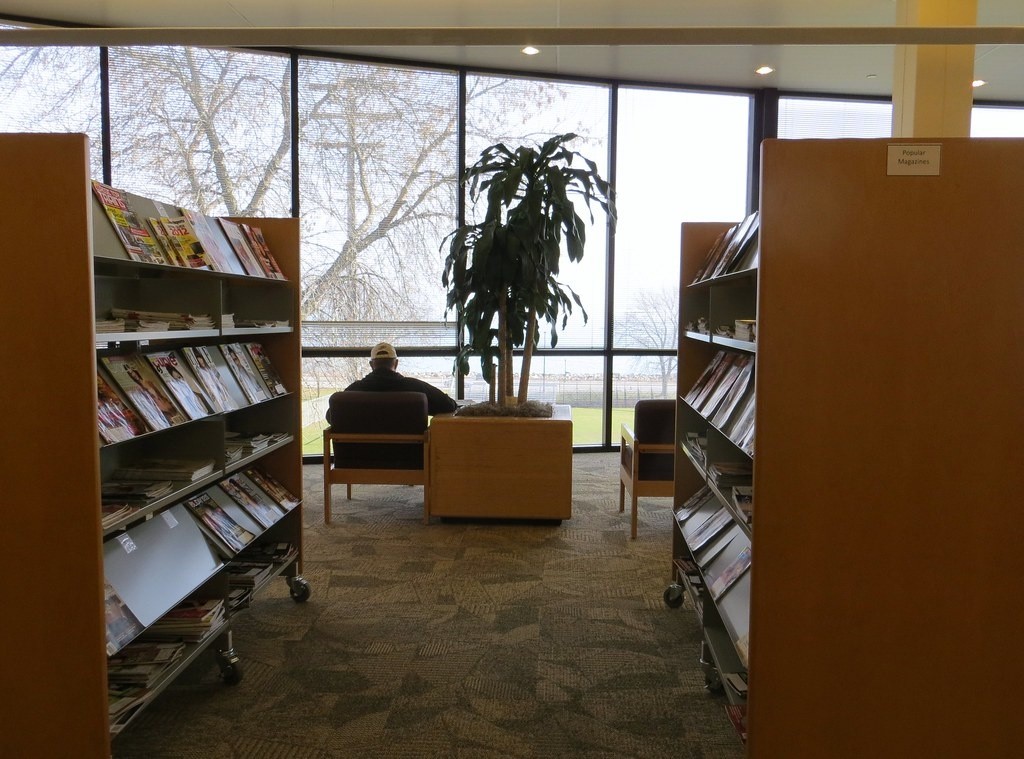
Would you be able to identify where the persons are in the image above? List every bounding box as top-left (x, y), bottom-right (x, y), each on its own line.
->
top-left (344, 341), bottom-right (459, 415)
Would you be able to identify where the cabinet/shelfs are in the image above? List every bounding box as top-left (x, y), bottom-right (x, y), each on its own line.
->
top-left (664, 137), bottom-right (1023, 759)
top-left (0, 132), bottom-right (310, 758)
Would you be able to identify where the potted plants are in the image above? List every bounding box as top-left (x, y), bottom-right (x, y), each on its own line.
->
top-left (431, 133), bottom-right (617, 523)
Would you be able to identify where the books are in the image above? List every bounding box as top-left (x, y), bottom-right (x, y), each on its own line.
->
top-left (685, 350), bottom-right (755, 458)
top-left (91, 180), bottom-right (286, 281)
top-left (94, 308), bottom-right (290, 334)
top-left (97, 337), bottom-right (290, 447)
top-left (687, 316), bottom-right (756, 343)
top-left (685, 429), bottom-right (752, 527)
top-left (689, 208), bottom-right (759, 284)
top-left (99, 428), bottom-right (290, 527)
top-left (674, 484), bottom-right (749, 699)
top-left (104, 463), bottom-right (301, 736)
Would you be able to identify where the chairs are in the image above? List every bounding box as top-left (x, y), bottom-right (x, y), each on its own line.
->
top-left (324, 391), bottom-right (430, 524)
top-left (619, 400), bottom-right (676, 539)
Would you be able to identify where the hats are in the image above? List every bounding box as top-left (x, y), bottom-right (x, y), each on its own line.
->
top-left (370, 342), bottom-right (397, 359)
